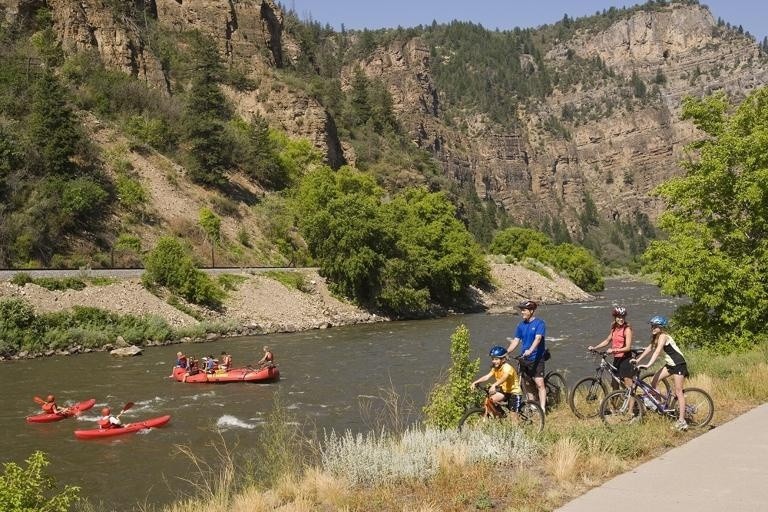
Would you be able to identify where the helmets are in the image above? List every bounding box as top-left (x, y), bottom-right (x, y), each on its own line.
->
top-left (517, 299), bottom-right (538, 311)
top-left (648, 315), bottom-right (668, 327)
top-left (611, 306), bottom-right (628, 317)
top-left (101, 408), bottom-right (110, 416)
top-left (47, 395), bottom-right (55, 403)
top-left (489, 346), bottom-right (508, 359)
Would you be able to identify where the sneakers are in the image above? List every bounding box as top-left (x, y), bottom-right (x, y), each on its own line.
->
top-left (488, 411), bottom-right (507, 418)
top-left (675, 420), bottom-right (688, 432)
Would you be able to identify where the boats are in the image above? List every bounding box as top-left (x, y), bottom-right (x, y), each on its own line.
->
top-left (75, 413), bottom-right (175, 440)
top-left (169, 360), bottom-right (279, 385)
top-left (22, 399), bottom-right (98, 423)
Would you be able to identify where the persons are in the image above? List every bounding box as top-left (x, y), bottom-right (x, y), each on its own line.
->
top-left (628, 315), bottom-right (689, 433)
top-left (587, 306), bottom-right (635, 422)
top-left (470, 345), bottom-right (524, 430)
top-left (507, 300), bottom-right (547, 414)
top-left (96, 407), bottom-right (132, 430)
top-left (168, 351), bottom-right (229, 383)
top-left (41, 394), bottom-right (69, 414)
top-left (258, 346), bottom-right (273, 371)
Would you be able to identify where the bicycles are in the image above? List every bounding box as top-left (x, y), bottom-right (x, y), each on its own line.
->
top-left (459, 344), bottom-right (716, 440)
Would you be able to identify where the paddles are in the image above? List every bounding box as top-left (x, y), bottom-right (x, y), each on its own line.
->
top-left (32, 395), bottom-right (46, 406)
top-left (116, 401), bottom-right (133, 420)
top-left (202, 363), bottom-right (208, 382)
